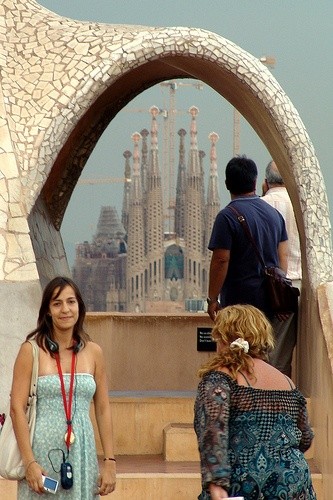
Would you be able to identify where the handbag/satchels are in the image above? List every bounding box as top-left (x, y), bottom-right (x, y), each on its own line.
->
top-left (0, 340), bottom-right (39, 480)
top-left (256, 263), bottom-right (298, 317)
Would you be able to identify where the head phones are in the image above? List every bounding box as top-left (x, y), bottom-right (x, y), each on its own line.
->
top-left (46, 335), bottom-right (82, 352)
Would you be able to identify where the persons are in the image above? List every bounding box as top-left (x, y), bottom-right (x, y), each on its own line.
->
top-left (207, 157), bottom-right (301, 379)
top-left (194, 305), bottom-right (317, 500)
top-left (259, 159), bottom-right (302, 292)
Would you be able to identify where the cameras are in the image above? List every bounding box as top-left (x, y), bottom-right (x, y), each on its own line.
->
top-left (41, 476), bottom-right (59, 494)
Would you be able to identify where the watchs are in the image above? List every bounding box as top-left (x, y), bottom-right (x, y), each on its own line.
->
top-left (207, 297), bottom-right (218, 305)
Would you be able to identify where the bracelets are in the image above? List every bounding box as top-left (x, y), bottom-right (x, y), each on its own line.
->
top-left (10, 276), bottom-right (117, 500)
top-left (104, 458), bottom-right (116, 462)
